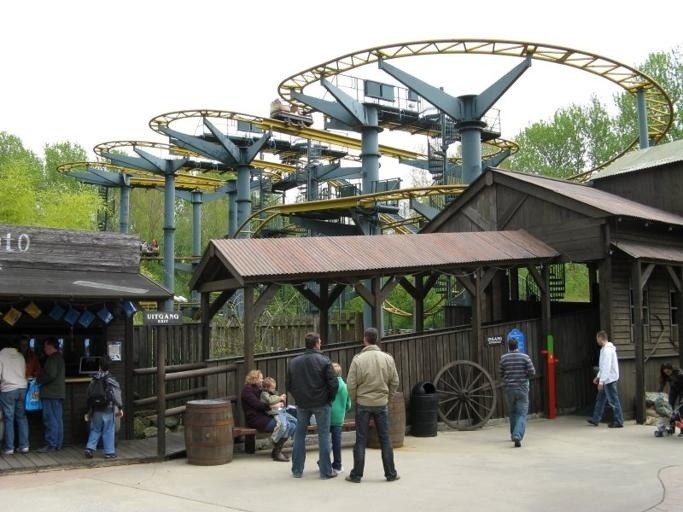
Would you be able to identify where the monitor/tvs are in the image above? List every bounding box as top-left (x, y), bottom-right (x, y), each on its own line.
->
top-left (79, 356), bottom-right (103, 374)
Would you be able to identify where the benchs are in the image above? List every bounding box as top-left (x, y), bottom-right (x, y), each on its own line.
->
top-left (232, 422), bottom-right (374, 454)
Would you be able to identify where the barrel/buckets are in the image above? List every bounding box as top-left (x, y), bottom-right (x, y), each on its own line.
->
top-left (184, 400), bottom-right (235, 465)
top-left (366, 391), bottom-right (406, 449)
top-left (413, 393), bottom-right (438, 438)
top-left (414, 381), bottom-right (436, 394)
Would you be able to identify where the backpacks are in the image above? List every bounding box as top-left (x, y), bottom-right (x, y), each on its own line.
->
top-left (88, 374), bottom-right (111, 411)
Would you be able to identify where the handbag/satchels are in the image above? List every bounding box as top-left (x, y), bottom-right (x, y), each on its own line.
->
top-left (26, 378), bottom-right (42, 411)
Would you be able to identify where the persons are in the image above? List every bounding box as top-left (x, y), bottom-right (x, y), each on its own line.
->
top-left (586, 330), bottom-right (624, 427)
top-left (346, 327), bottom-right (400, 483)
top-left (241, 333), bottom-right (353, 479)
top-left (18, 336), bottom-right (43, 378)
top-left (0, 338), bottom-right (30, 454)
top-left (36, 336), bottom-right (66, 453)
top-left (645, 361), bottom-right (683, 438)
top-left (301, 108), bottom-right (313, 128)
top-left (273, 100), bottom-right (291, 127)
top-left (290, 106), bottom-right (304, 128)
top-left (498, 338), bottom-right (536, 447)
top-left (140, 239), bottom-right (150, 261)
top-left (147, 239), bottom-right (159, 261)
top-left (84, 356), bottom-right (124, 459)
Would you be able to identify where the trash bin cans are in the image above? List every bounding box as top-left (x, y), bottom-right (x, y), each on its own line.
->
top-left (411, 381), bottom-right (439, 437)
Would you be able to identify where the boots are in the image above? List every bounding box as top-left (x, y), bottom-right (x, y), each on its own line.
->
top-left (271, 438), bottom-right (289, 461)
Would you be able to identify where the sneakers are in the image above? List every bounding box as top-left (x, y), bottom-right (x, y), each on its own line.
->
top-left (1, 448), bottom-right (14, 454)
top-left (16, 447), bottom-right (29, 453)
top-left (588, 419), bottom-right (598, 425)
top-left (36, 445), bottom-right (62, 453)
top-left (386, 476), bottom-right (400, 480)
top-left (609, 421), bottom-right (623, 428)
top-left (105, 454), bottom-right (117, 458)
top-left (667, 428), bottom-right (683, 437)
top-left (514, 437), bottom-right (521, 447)
top-left (85, 449), bottom-right (92, 458)
top-left (317, 460), bottom-right (361, 482)
